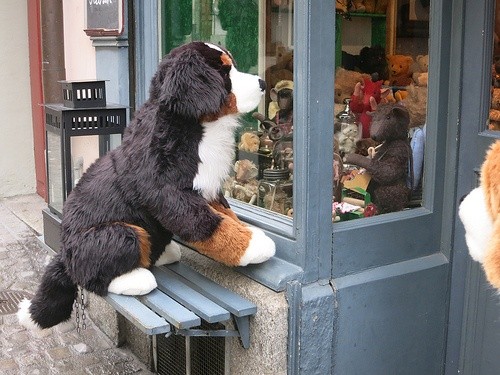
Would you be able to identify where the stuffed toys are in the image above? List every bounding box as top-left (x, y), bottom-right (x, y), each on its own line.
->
top-left (17, 40), bottom-right (276, 337)
top-left (218, 0), bottom-right (500, 224)
top-left (458, 138), bottom-right (500, 294)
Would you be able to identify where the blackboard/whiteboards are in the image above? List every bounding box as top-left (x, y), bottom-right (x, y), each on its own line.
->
top-left (84, 0), bottom-right (124, 35)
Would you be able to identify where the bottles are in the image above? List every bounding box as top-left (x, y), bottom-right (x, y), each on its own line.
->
top-left (259, 169), bottom-right (293, 215)
top-left (335, 99), bottom-right (358, 160)
